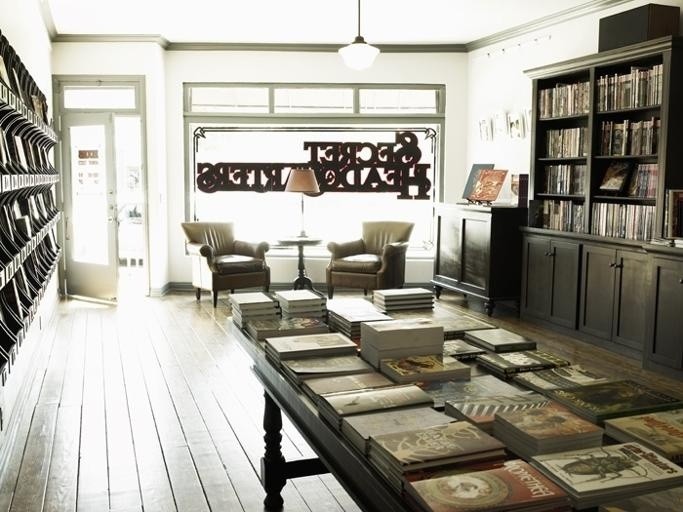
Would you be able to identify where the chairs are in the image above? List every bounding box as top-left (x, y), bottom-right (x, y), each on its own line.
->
top-left (178, 220), bottom-right (271, 308)
top-left (326, 220), bottom-right (415, 299)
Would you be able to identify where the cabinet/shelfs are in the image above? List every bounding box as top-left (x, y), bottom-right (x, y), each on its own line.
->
top-left (431, 198), bottom-right (521, 320)
top-left (0, 81), bottom-right (64, 424)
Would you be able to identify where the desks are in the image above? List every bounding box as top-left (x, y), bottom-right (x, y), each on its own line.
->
top-left (225, 314), bottom-right (679, 511)
top-left (277, 238), bottom-right (325, 297)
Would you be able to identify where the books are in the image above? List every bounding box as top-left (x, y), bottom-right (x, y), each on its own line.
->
top-left (229, 287), bottom-right (683, 512)
top-left (526, 64), bottom-right (683, 250)
top-left (0, 54), bottom-right (57, 322)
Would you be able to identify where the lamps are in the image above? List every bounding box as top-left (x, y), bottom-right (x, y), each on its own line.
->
top-left (336, 0), bottom-right (381, 72)
top-left (283, 168), bottom-right (321, 238)
